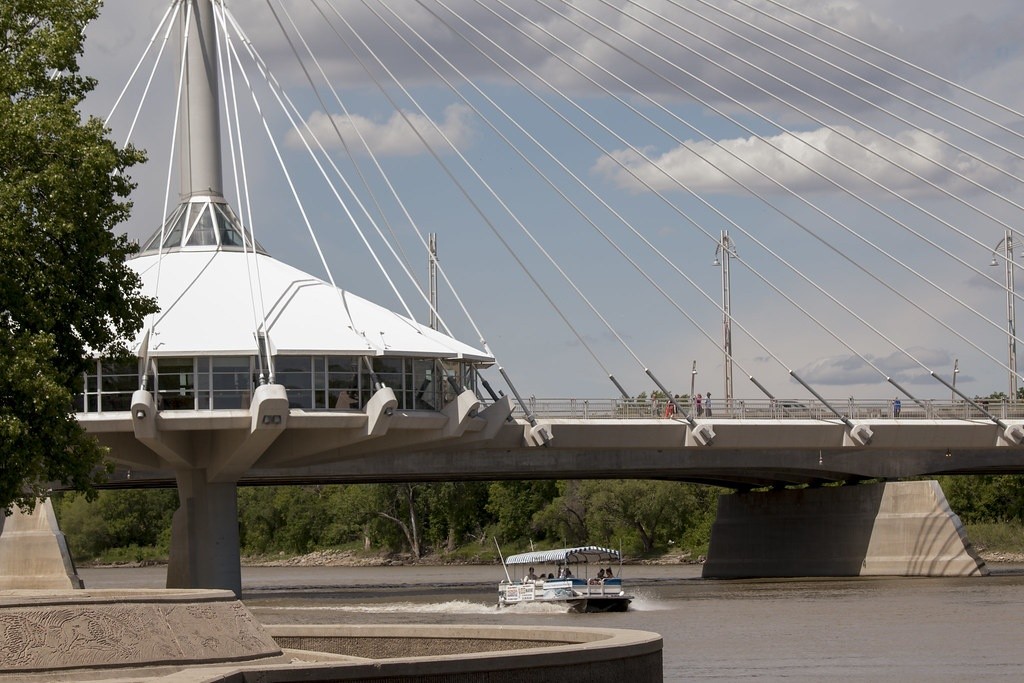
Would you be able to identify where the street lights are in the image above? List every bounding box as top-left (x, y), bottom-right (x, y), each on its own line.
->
top-left (713, 229), bottom-right (740, 417)
top-left (988, 226), bottom-right (1024, 413)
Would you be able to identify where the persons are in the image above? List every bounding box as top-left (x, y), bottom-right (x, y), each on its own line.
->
top-left (590, 568), bottom-right (615, 586)
top-left (696, 394), bottom-right (702, 417)
top-left (665, 394), bottom-right (679, 417)
top-left (705, 391), bottom-right (711, 417)
top-left (524, 567), bottom-right (571, 582)
top-left (894, 397), bottom-right (901, 417)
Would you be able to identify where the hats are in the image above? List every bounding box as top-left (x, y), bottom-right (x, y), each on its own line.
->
top-left (707, 393), bottom-right (711, 395)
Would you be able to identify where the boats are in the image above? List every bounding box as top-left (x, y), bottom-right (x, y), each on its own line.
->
top-left (497, 545), bottom-right (636, 609)
